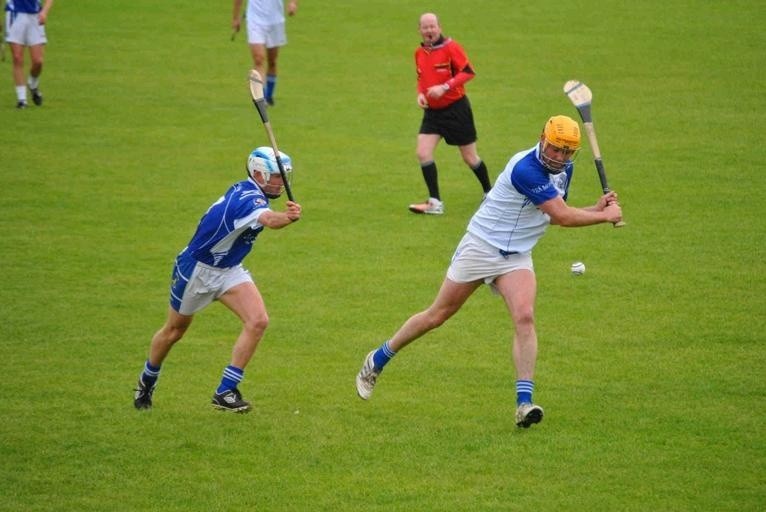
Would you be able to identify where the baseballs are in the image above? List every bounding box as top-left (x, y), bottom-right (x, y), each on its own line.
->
top-left (571, 261), bottom-right (585, 275)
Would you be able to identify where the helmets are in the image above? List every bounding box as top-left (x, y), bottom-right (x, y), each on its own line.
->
top-left (540, 115), bottom-right (583, 174)
top-left (246, 146), bottom-right (292, 199)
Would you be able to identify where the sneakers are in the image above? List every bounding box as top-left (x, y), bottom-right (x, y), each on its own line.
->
top-left (516, 402), bottom-right (543, 428)
top-left (16, 101), bottom-right (29, 109)
top-left (355, 349), bottom-right (384, 401)
top-left (27, 80), bottom-right (42, 106)
top-left (409, 197), bottom-right (445, 215)
top-left (211, 387), bottom-right (252, 415)
top-left (134, 375), bottom-right (157, 410)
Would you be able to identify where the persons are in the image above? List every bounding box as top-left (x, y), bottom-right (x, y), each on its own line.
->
top-left (407, 12), bottom-right (492, 216)
top-left (354, 114), bottom-right (623, 428)
top-left (231, 0), bottom-right (297, 106)
top-left (3, 0), bottom-right (53, 109)
top-left (132, 145), bottom-right (304, 413)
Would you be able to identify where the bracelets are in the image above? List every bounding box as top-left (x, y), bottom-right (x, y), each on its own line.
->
top-left (442, 81), bottom-right (451, 91)
